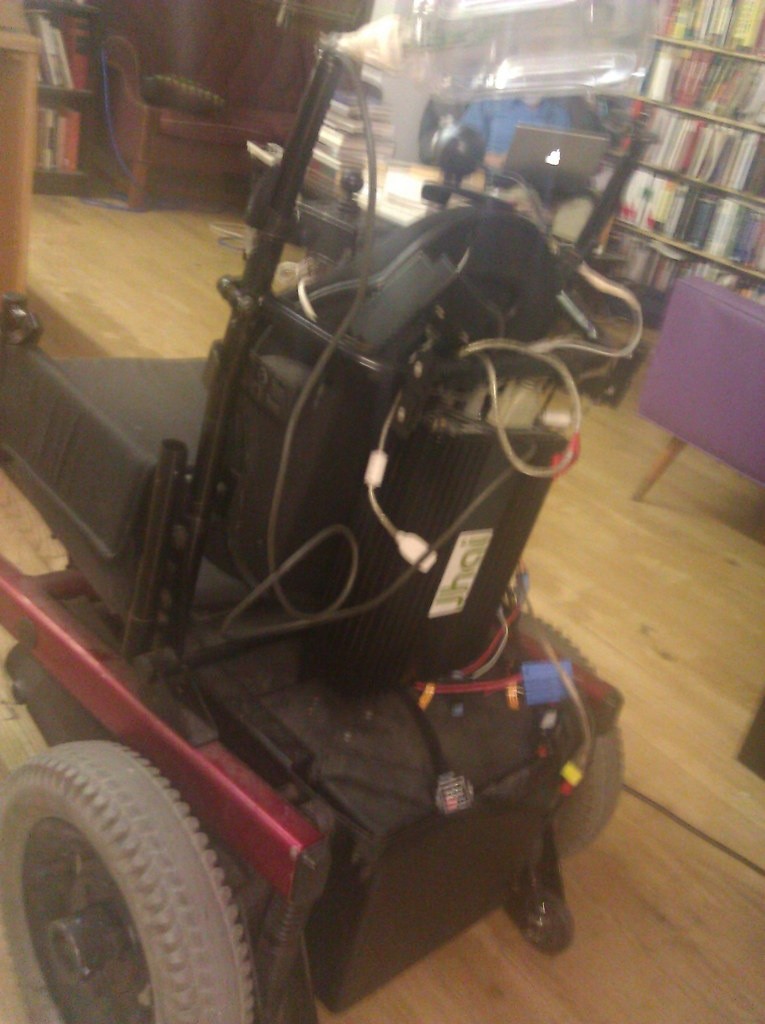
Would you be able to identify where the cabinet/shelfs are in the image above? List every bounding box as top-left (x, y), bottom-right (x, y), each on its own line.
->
top-left (596, 0), bottom-right (765, 339)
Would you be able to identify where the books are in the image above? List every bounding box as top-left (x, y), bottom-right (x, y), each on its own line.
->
top-left (29, 10), bottom-right (88, 89)
top-left (608, 1), bottom-right (765, 294)
top-left (37, 102), bottom-right (80, 175)
top-left (245, 91), bottom-right (458, 233)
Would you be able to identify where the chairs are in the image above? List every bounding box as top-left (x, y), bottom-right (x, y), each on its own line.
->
top-left (627, 274), bottom-right (765, 501)
top-left (418, 100), bottom-right (484, 204)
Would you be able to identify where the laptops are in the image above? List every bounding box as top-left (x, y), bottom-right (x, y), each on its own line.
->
top-left (495, 124), bottom-right (610, 192)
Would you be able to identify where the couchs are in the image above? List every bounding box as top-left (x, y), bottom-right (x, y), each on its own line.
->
top-left (91, 0), bottom-right (308, 218)
